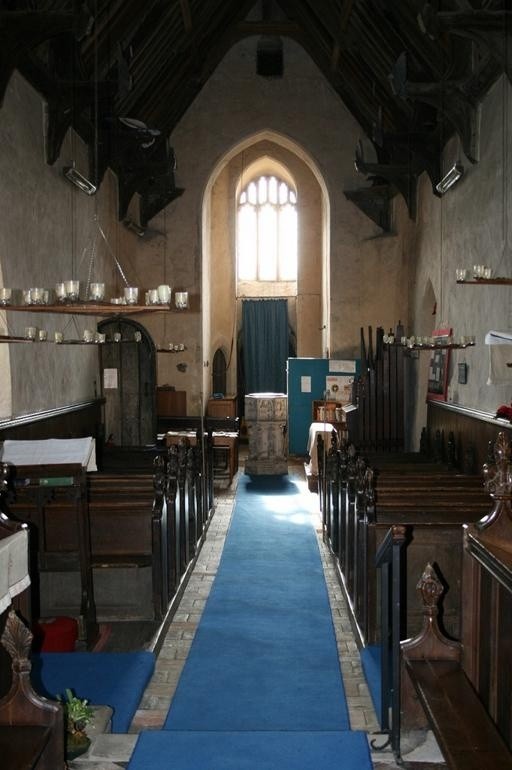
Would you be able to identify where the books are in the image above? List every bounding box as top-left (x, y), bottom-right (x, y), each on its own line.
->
top-left (1, 437), bottom-right (93, 468)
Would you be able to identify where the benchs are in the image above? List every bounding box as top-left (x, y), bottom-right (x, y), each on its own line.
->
top-left (318, 427), bottom-right (512, 770)
top-left (0, 412), bottom-right (215, 623)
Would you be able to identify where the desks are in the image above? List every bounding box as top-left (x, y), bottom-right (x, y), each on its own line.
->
top-left (167, 429), bottom-right (238, 483)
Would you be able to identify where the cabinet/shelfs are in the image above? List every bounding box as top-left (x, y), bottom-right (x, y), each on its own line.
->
top-left (209, 395), bottom-right (238, 420)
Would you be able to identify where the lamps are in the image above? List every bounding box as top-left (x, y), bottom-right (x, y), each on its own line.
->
top-left (381, 326), bottom-right (478, 350)
top-left (0, 0), bottom-right (191, 353)
top-left (454, 263), bottom-right (512, 286)
top-left (435, 159), bottom-right (464, 196)
